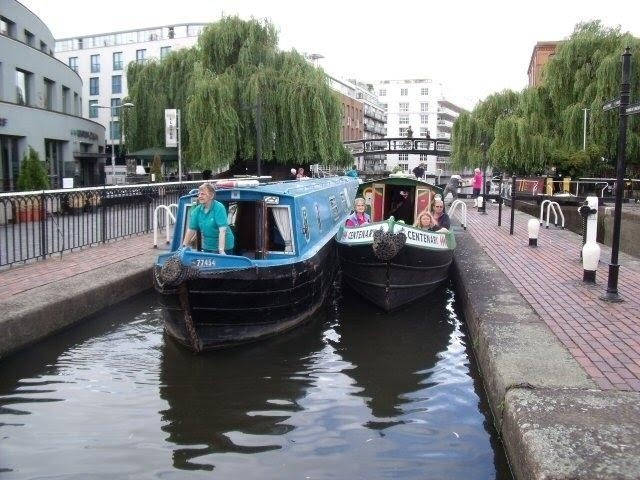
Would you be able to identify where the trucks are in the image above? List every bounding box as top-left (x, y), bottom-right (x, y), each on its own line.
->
top-left (104, 164), bottom-right (146, 185)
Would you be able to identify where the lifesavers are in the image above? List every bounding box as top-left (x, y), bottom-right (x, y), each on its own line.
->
top-left (216, 179), bottom-right (260, 189)
top-left (389, 174), bottom-right (418, 181)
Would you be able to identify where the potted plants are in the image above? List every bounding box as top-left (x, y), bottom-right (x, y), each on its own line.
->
top-left (10, 142), bottom-right (55, 225)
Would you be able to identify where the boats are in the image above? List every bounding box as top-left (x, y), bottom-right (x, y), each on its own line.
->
top-left (151, 174), bottom-right (362, 351)
top-left (336, 173), bottom-right (457, 313)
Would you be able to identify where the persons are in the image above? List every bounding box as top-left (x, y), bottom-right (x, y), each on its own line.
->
top-left (470, 167), bottom-right (482, 209)
top-left (402, 126), bottom-right (414, 149)
top-left (295, 168), bottom-right (305, 178)
top-left (426, 130), bottom-right (431, 149)
top-left (287, 168), bottom-right (297, 180)
top-left (344, 165), bottom-right (358, 177)
top-left (414, 212), bottom-right (451, 234)
top-left (345, 197), bottom-right (372, 227)
top-left (183, 183), bottom-right (236, 254)
top-left (427, 198), bottom-right (450, 230)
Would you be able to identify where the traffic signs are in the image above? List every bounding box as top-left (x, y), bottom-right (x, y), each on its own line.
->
top-left (625, 103), bottom-right (640, 115)
top-left (602, 98), bottom-right (620, 111)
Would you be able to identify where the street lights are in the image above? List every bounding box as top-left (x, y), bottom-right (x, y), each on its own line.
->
top-left (91, 101), bottom-right (136, 185)
top-left (481, 133), bottom-right (490, 215)
top-left (581, 107), bottom-right (591, 152)
